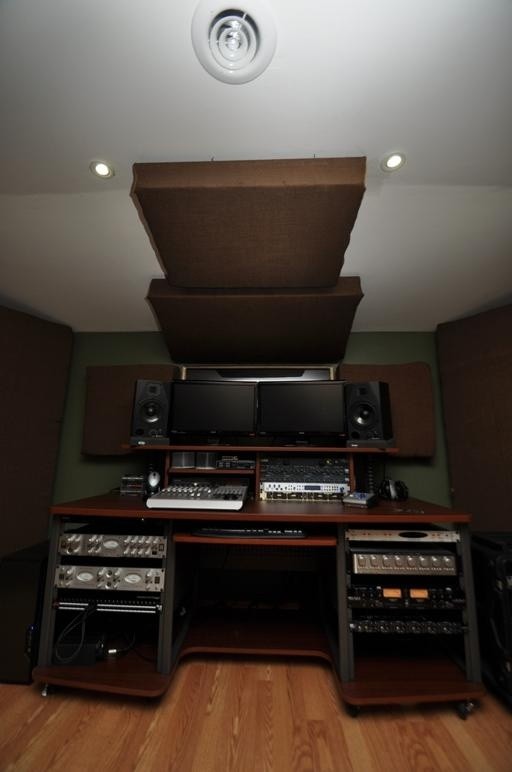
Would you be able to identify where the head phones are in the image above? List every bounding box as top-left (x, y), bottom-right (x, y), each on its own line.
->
top-left (378, 479), bottom-right (408, 501)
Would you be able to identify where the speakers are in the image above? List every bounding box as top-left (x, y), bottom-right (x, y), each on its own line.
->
top-left (131, 378), bottom-right (170, 447)
top-left (345, 381), bottom-right (396, 448)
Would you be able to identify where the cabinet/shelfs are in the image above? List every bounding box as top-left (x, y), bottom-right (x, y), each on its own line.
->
top-left (335, 356), bottom-right (437, 460)
top-left (430, 294), bottom-right (511, 531)
top-left (81, 362), bottom-right (170, 461)
top-left (0, 301), bottom-right (80, 556)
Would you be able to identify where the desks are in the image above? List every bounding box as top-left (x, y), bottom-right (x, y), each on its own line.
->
top-left (31, 485), bottom-right (485, 722)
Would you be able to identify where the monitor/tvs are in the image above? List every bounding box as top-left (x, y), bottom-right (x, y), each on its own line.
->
top-left (168, 379), bottom-right (257, 445)
top-left (258, 381), bottom-right (346, 447)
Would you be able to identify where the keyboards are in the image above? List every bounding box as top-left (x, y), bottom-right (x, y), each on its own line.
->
top-left (191, 525), bottom-right (307, 538)
